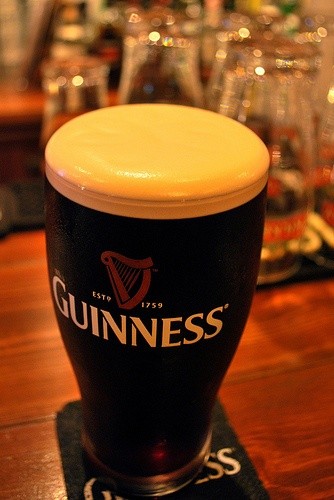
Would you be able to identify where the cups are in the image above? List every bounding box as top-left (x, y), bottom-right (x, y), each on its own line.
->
top-left (222, 48), bottom-right (320, 282)
top-left (42, 101), bottom-right (269, 496)
top-left (42, 2), bottom-right (325, 126)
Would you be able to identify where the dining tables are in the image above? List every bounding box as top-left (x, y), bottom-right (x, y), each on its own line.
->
top-left (0, 225), bottom-right (333, 500)
